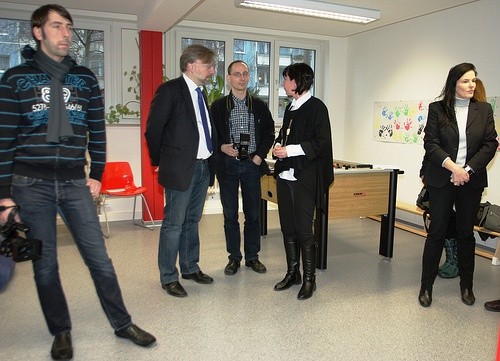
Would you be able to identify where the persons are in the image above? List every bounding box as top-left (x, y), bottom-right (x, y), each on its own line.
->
top-left (272, 63), bottom-right (334, 300)
top-left (145, 44), bottom-right (215, 297)
top-left (0, 4), bottom-right (156, 361)
top-left (418, 63), bottom-right (500, 312)
top-left (210, 60), bottom-right (276, 275)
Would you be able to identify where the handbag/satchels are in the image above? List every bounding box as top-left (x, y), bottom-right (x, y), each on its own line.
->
top-left (474, 200), bottom-right (500, 242)
top-left (416, 186), bottom-right (430, 212)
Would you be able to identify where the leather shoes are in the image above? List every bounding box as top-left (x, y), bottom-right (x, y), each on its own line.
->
top-left (51, 331), bottom-right (73, 361)
top-left (114, 324), bottom-right (156, 346)
top-left (461, 287), bottom-right (475, 305)
top-left (245, 259), bottom-right (266, 273)
top-left (162, 281), bottom-right (187, 297)
top-left (419, 287), bottom-right (432, 307)
top-left (484, 300), bottom-right (500, 312)
top-left (224, 259), bottom-right (240, 275)
top-left (182, 270), bottom-right (213, 284)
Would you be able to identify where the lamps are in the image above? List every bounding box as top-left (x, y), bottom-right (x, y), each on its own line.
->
top-left (234, 0), bottom-right (380, 24)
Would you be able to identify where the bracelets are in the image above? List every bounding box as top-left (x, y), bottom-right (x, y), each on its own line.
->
top-left (462, 164), bottom-right (473, 174)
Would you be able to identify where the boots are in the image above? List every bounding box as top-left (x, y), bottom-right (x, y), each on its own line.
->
top-left (438, 238), bottom-right (459, 278)
top-left (298, 244), bottom-right (316, 300)
top-left (274, 238), bottom-right (302, 291)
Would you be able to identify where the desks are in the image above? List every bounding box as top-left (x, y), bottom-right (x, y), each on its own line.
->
top-left (261, 158), bottom-right (404, 270)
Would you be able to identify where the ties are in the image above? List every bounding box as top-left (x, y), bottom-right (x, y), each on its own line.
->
top-left (195, 86), bottom-right (214, 154)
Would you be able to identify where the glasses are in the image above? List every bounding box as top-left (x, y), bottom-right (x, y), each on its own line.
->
top-left (229, 72), bottom-right (249, 78)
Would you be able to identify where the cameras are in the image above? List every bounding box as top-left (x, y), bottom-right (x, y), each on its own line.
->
top-left (234, 132), bottom-right (250, 161)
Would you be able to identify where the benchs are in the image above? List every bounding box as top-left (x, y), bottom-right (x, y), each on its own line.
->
top-left (369, 201), bottom-right (500, 265)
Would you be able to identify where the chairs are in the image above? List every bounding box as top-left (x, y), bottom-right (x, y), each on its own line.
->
top-left (98, 161), bottom-right (156, 238)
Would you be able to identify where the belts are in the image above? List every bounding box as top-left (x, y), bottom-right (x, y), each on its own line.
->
top-left (220, 153), bottom-right (255, 161)
